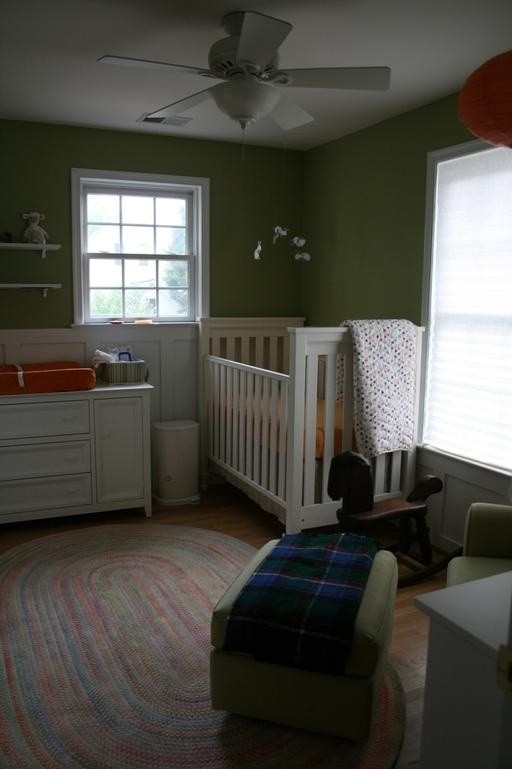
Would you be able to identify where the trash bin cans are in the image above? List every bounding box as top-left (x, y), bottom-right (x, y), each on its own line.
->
top-left (151, 417), bottom-right (203, 505)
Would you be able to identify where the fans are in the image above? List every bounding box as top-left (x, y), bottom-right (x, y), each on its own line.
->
top-left (96, 11), bottom-right (391, 131)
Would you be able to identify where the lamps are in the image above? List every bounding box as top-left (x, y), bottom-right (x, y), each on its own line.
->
top-left (210, 79), bottom-right (284, 139)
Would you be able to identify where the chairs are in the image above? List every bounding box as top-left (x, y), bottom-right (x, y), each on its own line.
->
top-left (446, 502), bottom-right (512, 587)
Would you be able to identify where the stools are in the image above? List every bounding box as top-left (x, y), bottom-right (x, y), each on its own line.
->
top-left (209, 534), bottom-right (400, 745)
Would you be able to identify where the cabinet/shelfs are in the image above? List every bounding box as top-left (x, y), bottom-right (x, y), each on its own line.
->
top-left (1, 383), bottom-right (154, 523)
top-left (0, 242), bottom-right (64, 299)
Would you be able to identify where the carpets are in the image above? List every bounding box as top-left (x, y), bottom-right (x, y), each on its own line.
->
top-left (1, 525), bottom-right (406, 768)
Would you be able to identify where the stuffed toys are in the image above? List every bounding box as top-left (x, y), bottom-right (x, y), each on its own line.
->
top-left (24, 210), bottom-right (51, 244)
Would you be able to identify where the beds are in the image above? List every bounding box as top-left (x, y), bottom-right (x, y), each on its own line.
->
top-left (194, 316), bottom-right (426, 534)
